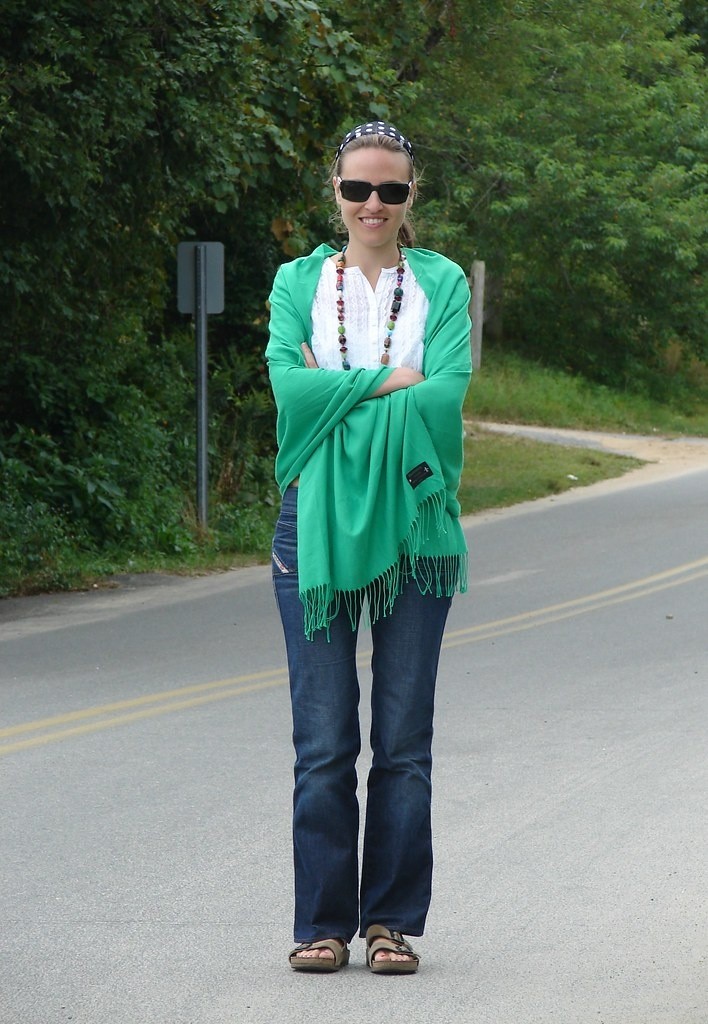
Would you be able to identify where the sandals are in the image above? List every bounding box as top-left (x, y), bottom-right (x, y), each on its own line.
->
top-left (365, 925), bottom-right (420, 972)
top-left (287, 937), bottom-right (350, 971)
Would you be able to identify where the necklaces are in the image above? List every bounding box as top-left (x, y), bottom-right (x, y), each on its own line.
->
top-left (336, 245), bottom-right (405, 371)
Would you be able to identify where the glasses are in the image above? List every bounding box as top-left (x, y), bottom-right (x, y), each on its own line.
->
top-left (337, 177), bottom-right (414, 205)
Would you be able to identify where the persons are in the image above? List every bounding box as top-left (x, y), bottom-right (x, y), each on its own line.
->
top-left (265, 122), bottom-right (473, 972)
